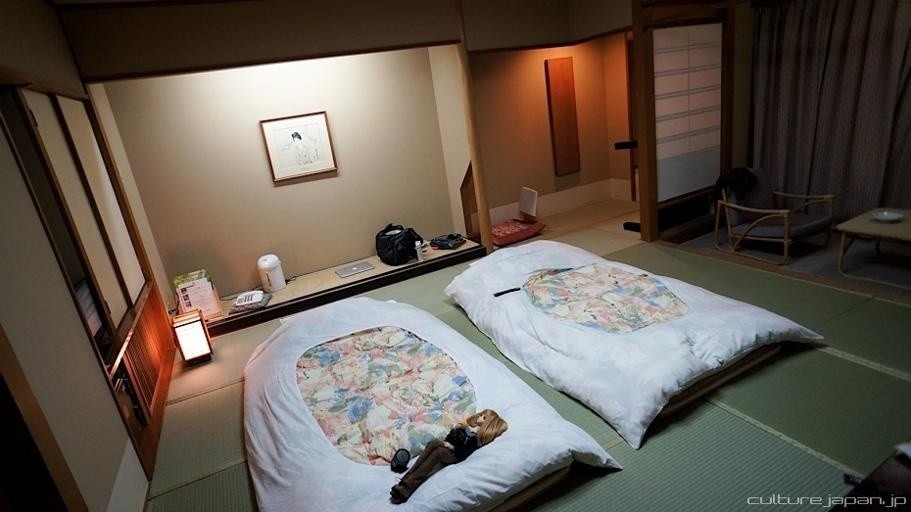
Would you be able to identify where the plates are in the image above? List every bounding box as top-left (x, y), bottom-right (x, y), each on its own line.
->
top-left (873, 211), bottom-right (904, 222)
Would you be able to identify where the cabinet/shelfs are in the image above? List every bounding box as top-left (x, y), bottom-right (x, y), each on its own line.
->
top-left (34, 88), bottom-right (174, 481)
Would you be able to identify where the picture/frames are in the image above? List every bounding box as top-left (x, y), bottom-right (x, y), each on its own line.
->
top-left (259, 110), bottom-right (338, 189)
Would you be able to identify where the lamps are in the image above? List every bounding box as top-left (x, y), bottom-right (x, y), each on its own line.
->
top-left (171, 310), bottom-right (215, 366)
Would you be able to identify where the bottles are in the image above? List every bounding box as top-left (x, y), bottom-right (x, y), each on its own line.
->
top-left (415, 240), bottom-right (425, 262)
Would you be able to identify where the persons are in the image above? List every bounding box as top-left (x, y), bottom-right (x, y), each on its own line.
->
top-left (386, 406), bottom-right (507, 504)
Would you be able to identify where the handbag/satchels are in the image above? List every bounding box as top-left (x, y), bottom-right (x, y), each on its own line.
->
top-left (375, 222), bottom-right (424, 267)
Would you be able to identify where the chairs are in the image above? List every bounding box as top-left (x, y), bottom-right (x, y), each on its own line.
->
top-left (490, 184), bottom-right (547, 246)
top-left (712, 167), bottom-right (836, 263)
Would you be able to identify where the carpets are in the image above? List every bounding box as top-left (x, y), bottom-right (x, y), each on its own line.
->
top-left (686, 217), bottom-right (910, 300)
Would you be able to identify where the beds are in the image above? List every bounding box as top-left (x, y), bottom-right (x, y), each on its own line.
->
top-left (233, 291), bottom-right (622, 512)
top-left (445, 237), bottom-right (827, 454)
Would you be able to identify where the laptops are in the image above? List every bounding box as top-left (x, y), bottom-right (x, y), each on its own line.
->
top-left (335, 262), bottom-right (375, 278)
